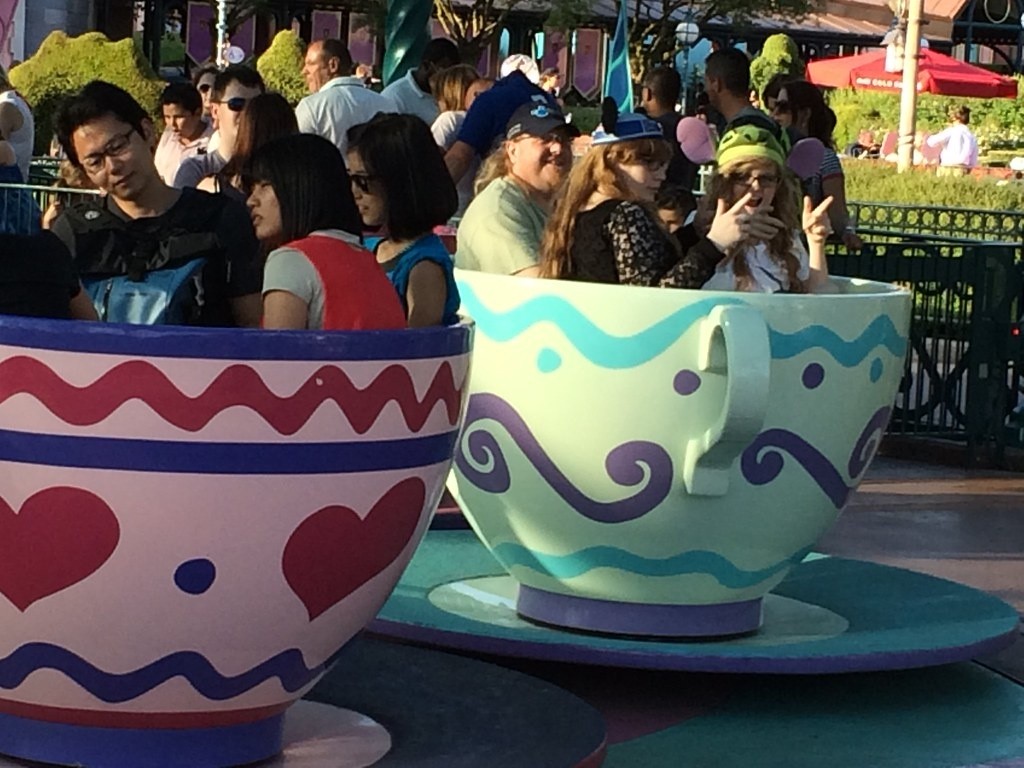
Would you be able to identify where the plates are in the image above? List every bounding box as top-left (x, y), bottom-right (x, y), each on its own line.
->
top-left (219, 637), bottom-right (609, 768)
top-left (366, 529), bottom-right (1018, 676)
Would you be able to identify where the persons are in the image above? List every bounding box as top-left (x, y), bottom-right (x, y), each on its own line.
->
top-left (0, 38), bottom-right (977, 329)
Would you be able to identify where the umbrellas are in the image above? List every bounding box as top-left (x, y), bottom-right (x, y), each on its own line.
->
top-left (807, 46), bottom-right (1020, 99)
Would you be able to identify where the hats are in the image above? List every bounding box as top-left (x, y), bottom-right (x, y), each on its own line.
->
top-left (675, 114), bottom-right (828, 181)
top-left (500, 52), bottom-right (540, 86)
top-left (588, 95), bottom-right (666, 145)
top-left (505, 98), bottom-right (581, 137)
top-left (722, 106), bottom-right (783, 140)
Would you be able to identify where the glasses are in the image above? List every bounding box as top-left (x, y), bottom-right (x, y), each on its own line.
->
top-left (556, 74), bottom-right (564, 80)
top-left (643, 156), bottom-right (669, 171)
top-left (733, 171), bottom-right (780, 189)
top-left (518, 131), bottom-right (575, 145)
top-left (79, 127), bottom-right (139, 176)
top-left (221, 96), bottom-right (248, 112)
top-left (346, 167), bottom-right (374, 193)
top-left (199, 82), bottom-right (213, 93)
top-left (774, 100), bottom-right (790, 114)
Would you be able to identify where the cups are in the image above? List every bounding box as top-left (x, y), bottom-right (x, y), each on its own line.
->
top-left (444, 270), bottom-right (913, 639)
top-left (1, 310), bottom-right (472, 768)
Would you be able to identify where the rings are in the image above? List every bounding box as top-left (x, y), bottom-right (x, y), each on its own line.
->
top-left (742, 213), bottom-right (750, 222)
